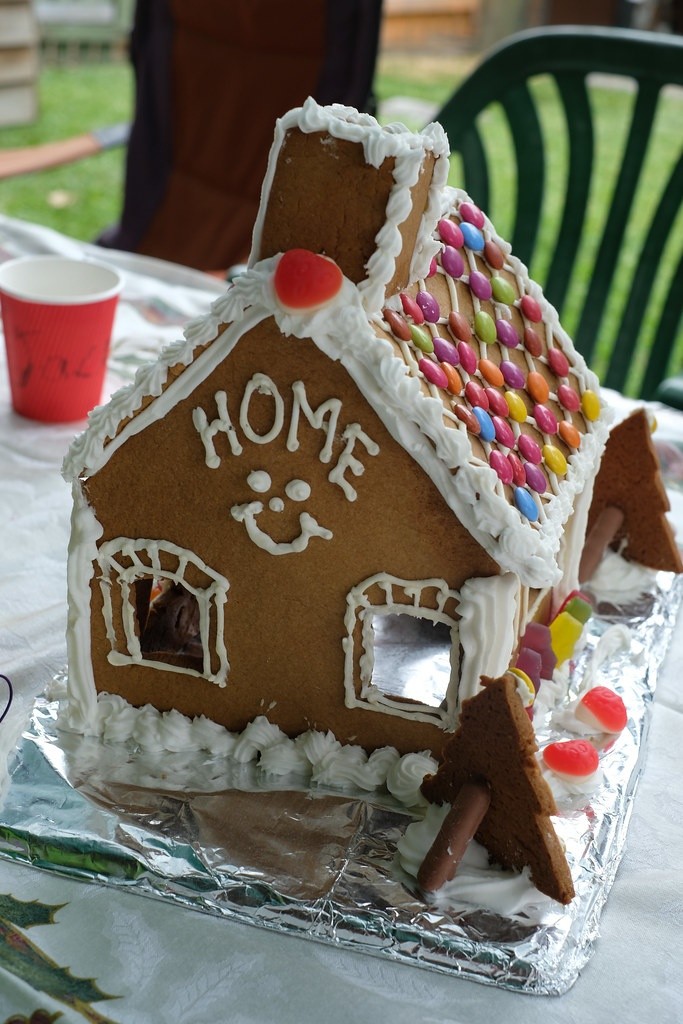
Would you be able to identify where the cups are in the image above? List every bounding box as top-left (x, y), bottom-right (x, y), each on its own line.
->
top-left (0, 254), bottom-right (121, 425)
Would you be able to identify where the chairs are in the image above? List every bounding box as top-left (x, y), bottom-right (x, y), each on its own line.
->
top-left (426, 24), bottom-right (683, 417)
